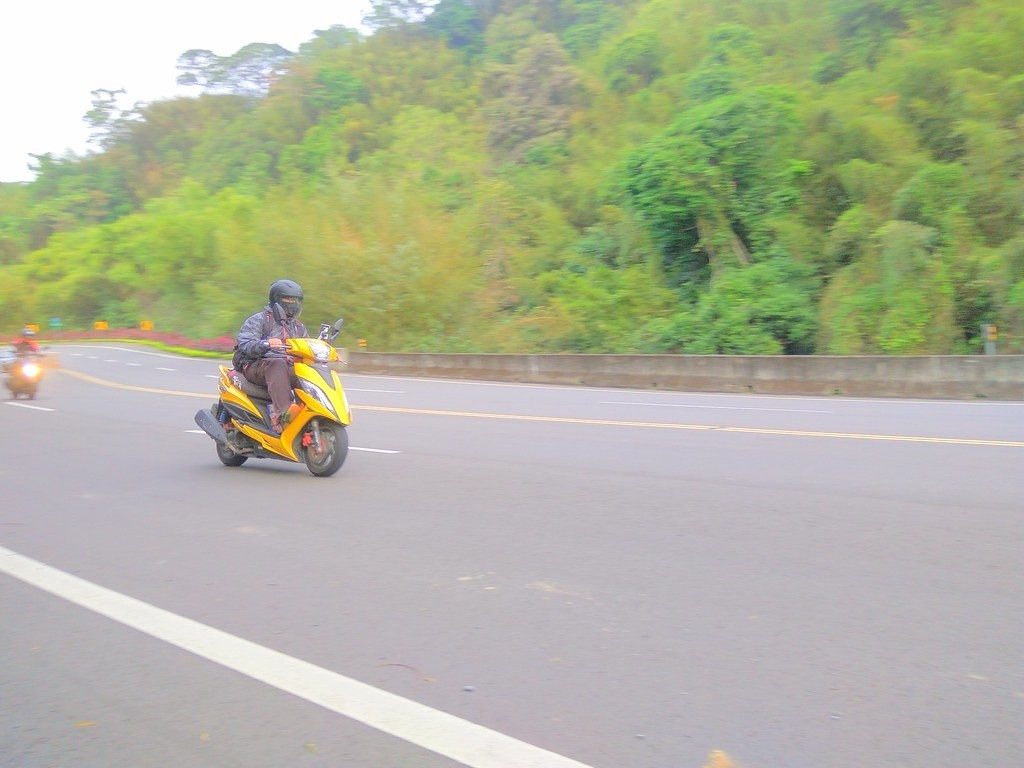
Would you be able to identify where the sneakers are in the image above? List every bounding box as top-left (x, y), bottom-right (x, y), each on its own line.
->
top-left (283, 404), bottom-right (301, 431)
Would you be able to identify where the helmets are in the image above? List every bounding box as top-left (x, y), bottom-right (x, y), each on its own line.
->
top-left (268, 279), bottom-right (303, 318)
top-left (23, 329), bottom-right (34, 335)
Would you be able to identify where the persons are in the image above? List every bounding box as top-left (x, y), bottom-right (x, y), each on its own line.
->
top-left (11, 329), bottom-right (38, 355)
top-left (231, 279), bottom-right (343, 420)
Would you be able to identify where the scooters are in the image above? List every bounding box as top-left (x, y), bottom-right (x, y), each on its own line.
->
top-left (194, 302), bottom-right (353, 477)
top-left (4, 345), bottom-right (48, 401)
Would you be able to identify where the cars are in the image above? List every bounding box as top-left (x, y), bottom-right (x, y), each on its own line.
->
top-left (0, 349), bottom-right (18, 373)
